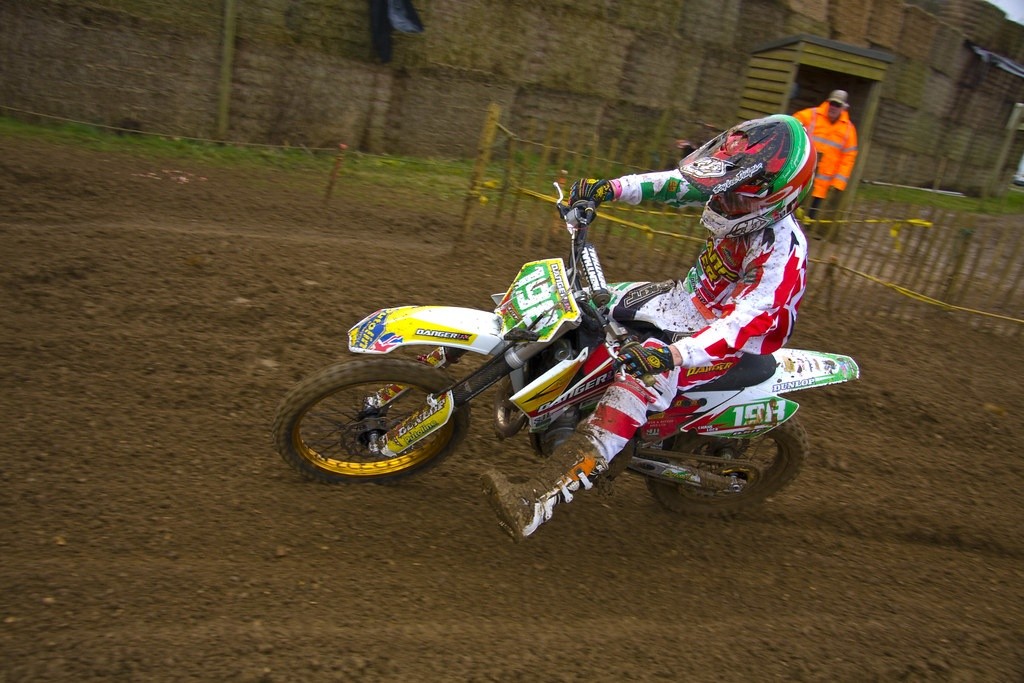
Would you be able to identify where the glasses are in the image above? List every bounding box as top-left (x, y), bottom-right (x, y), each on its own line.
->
top-left (830, 101), bottom-right (842, 107)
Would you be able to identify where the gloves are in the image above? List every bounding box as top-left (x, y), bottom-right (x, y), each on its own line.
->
top-left (613, 338), bottom-right (676, 378)
top-left (568, 178), bottom-right (614, 207)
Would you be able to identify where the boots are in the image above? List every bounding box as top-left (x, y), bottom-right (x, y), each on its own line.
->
top-left (481, 431), bottom-right (609, 544)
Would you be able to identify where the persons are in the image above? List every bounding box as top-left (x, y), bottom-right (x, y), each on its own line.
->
top-left (477, 111), bottom-right (809, 546)
top-left (789, 88), bottom-right (859, 232)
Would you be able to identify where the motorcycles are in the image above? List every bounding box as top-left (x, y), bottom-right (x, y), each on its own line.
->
top-left (272, 175), bottom-right (862, 522)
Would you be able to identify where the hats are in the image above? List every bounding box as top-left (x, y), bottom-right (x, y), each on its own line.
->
top-left (827, 90), bottom-right (850, 108)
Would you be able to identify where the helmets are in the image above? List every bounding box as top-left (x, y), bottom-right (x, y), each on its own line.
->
top-left (676, 114), bottom-right (817, 238)
